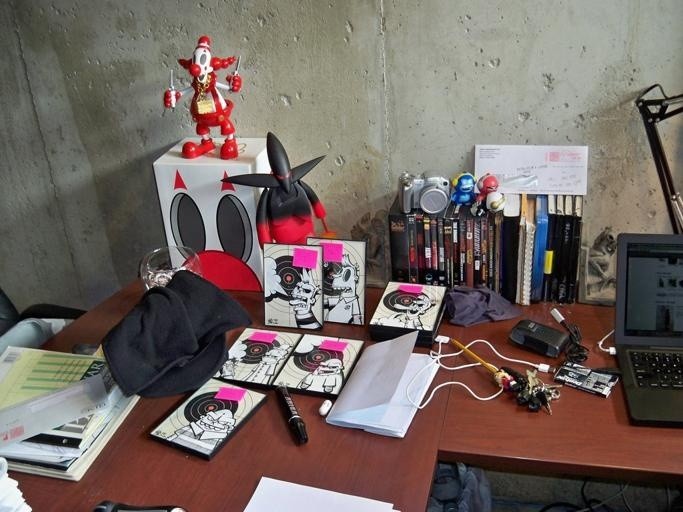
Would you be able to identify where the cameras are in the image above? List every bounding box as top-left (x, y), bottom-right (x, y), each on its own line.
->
top-left (398, 170), bottom-right (451, 215)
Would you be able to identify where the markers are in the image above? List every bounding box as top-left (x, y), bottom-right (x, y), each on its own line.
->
top-left (277, 386), bottom-right (308, 444)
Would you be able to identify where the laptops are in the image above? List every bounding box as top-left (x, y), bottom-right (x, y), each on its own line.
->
top-left (614, 233), bottom-right (683, 429)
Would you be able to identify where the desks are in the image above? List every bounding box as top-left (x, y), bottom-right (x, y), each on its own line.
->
top-left (435, 291), bottom-right (682, 488)
top-left (1, 266), bottom-right (465, 512)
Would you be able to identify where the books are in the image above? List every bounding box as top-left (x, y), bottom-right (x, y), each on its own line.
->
top-left (390, 190), bottom-right (586, 308)
top-left (326, 330), bottom-right (441, 439)
top-left (242, 473), bottom-right (401, 512)
top-left (0, 345), bottom-right (142, 485)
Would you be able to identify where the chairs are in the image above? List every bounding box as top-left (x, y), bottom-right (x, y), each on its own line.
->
top-left (0, 286), bottom-right (89, 340)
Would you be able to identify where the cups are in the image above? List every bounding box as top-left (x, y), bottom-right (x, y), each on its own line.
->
top-left (138, 243), bottom-right (201, 292)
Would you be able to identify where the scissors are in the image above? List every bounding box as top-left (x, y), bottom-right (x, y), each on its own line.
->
top-left (229, 55), bottom-right (241, 89)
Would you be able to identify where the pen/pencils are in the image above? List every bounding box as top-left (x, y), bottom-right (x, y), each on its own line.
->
top-left (450, 338), bottom-right (517, 387)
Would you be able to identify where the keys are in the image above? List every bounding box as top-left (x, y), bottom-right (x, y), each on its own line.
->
top-left (509, 369), bottom-right (565, 417)
